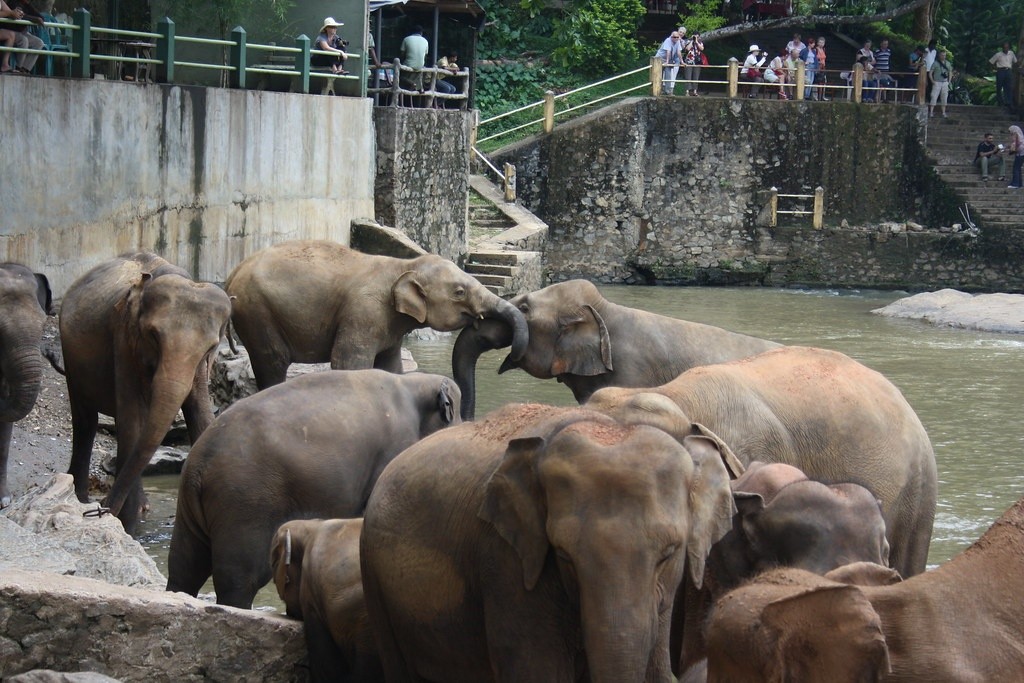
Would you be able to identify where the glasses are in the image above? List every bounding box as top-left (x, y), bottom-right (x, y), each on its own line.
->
top-left (672, 36), bottom-right (680, 38)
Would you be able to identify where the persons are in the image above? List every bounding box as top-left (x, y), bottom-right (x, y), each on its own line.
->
top-left (974, 132), bottom-right (1006, 182)
top-left (904, 39), bottom-right (938, 104)
top-left (400, 26), bottom-right (429, 92)
top-left (989, 42), bottom-right (1018, 106)
top-left (0, 0), bottom-right (65, 77)
top-left (784, 33), bottom-right (827, 101)
top-left (928, 50), bottom-right (953, 119)
top-left (849, 39), bottom-right (891, 103)
top-left (764, 49), bottom-right (789, 100)
top-left (423, 51), bottom-right (460, 110)
top-left (310, 16), bottom-right (350, 75)
top-left (1007, 125), bottom-right (1024, 189)
top-left (740, 44), bottom-right (768, 99)
top-left (655, 26), bottom-right (704, 96)
top-left (368, 32), bottom-right (381, 76)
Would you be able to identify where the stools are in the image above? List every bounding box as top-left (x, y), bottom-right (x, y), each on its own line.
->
top-left (118, 41), bottom-right (157, 82)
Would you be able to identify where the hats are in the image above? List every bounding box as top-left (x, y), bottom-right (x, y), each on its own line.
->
top-left (318, 16), bottom-right (346, 33)
top-left (748, 44), bottom-right (761, 53)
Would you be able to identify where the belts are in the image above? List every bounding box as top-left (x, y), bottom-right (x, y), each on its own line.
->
top-left (997, 67), bottom-right (1013, 71)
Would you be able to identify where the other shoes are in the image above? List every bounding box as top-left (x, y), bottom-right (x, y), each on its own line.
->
top-left (16, 66), bottom-right (33, 76)
top-left (685, 91), bottom-right (691, 97)
top-left (334, 70), bottom-right (350, 75)
top-left (998, 177), bottom-right (1009, 181)
top-left (418, 89), bottom-right (426, 94)
top-left (805, 96), bottom-right (829, 100)
top-left (1007, 185), bottom-right (1022, 189)
top-left (409, 82), bottom-right (418, 92)
top-left (748, 94), bottom-right (760, 98)
top-left (667, 92), bottom-right (675, 96)
top-left (693, 93), bottom-right (701, 97)
top-left (863, 97), bottom-right (890, 103)
top-left (778, 90), bottom-right (789, 100)
top-left (940, 112), bottom-right (948, 118)
top-left (982, 177), bottom-right (988, 181)
top-left (928, 112), bottom-right (935, 118)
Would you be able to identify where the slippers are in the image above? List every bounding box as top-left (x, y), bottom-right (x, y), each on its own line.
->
top-left (0, 66), bottom-right (23, 76)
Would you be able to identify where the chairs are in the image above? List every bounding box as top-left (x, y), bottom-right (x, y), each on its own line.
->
top-left (28, 12), bottom-right (72, 77)
top-left (839, 72), bottom-right (853, 101)
top-left (738, 67), bottom-right (834, 101)
top-left (368, 62), bottom-right (445, 109)
top-left (873, 74), bottom-right (898, 105)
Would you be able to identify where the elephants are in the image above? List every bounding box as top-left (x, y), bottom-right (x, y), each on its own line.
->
top-left (452, 278), bottom-right (784, 420)
top-left (223, 241), bottom-right (529, 393)
top-left (166, 368), bottom-right (463, 609)
top-left (700, 462), bottom-right (891, 586)
top-left (702, 500), bottom-right (1024, 683)
top-left (582, 348), bottom-right (937, 584)
top-left (40, 249), bottom-right (232, 536)
top-left (270, 518), bottom-right (377, 683)
top-left (358, 405), bottom-right (737, 683)
top-left (0, 261), bottom-right (58, 505)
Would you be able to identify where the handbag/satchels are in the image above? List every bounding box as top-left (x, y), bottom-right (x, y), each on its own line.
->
top-left (695, 54), bottom-right (704, 64)
top-left (701, 52), bottom-right (709, 65)
top-left (748, 63), bottom-right (763, 77)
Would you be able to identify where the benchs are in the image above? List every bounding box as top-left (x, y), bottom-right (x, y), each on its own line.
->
top-left (250, 41), bottom-right (338, 95)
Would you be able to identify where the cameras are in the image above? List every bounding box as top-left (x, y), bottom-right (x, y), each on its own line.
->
top-left (335, 38), bottom-right (350, 47)
top-left (941, 72), bottom-right (948, 78)
top-left (692, 36), bottom-right (696, 40)
